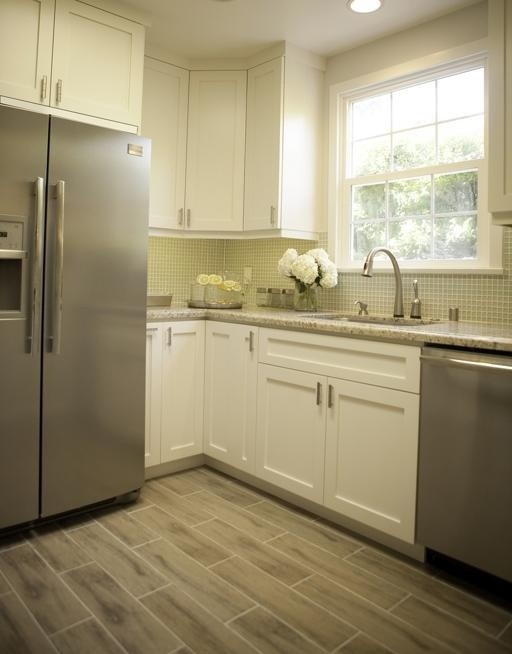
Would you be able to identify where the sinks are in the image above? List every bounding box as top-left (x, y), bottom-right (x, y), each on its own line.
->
top-left (299, 311), bottom-right (438, 325)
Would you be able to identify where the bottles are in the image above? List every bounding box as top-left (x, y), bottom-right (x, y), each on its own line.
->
top-left (223, 270), bottom-right (249, 306)
top-left (255, 287), bottom-right (294, 309)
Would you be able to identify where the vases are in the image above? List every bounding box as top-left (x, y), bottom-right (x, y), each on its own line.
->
top-left (294, 283), bottom-right (318, 310)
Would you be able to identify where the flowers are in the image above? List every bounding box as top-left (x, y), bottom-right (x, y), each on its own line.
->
top-left (278, 246), bottom-right (340, 310)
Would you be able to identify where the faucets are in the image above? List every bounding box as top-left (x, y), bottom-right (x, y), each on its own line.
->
top-left (362, 247), bottom-right (404, 319)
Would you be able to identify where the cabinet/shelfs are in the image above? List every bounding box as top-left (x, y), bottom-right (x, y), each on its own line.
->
top-left (146, 56), bottom-right (243, 237)
top-left (261, 327), bottom-right (422, 566)
top-left (0, 1), bottom-right (146, 137)
top-left (146, 320), bottom-right (259, 484)
top-left (487, 2), bottom-right (510, 223)
top-left (245, 56), bottom-right (324, 239)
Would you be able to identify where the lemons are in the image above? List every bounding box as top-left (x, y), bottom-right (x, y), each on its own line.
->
top-left (196, 274), bottom-right (241, 292)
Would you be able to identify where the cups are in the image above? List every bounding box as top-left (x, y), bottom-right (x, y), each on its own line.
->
top-left (190, 283), bottom-right (233, 306)
